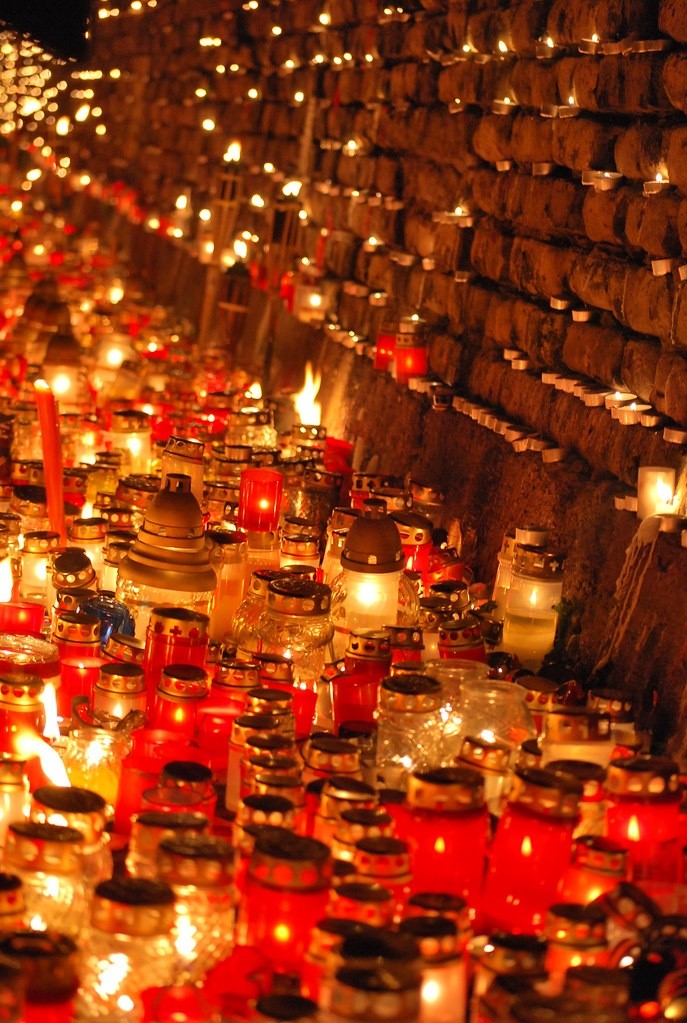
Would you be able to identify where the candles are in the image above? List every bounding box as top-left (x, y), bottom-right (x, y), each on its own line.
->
top-left (0, 0), bottom-right (687, 1023)
